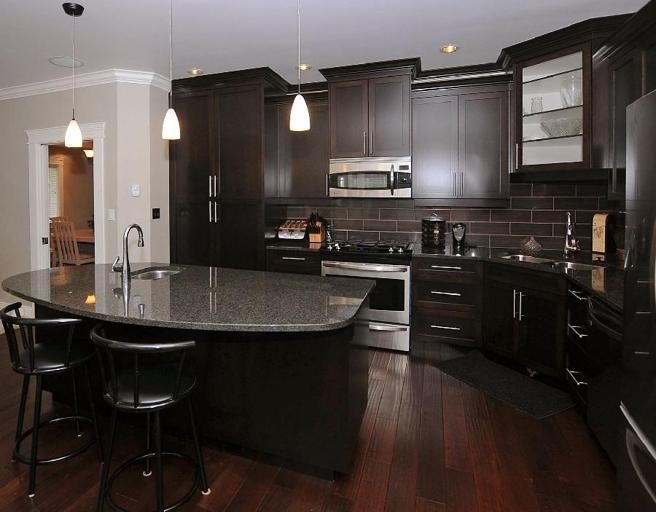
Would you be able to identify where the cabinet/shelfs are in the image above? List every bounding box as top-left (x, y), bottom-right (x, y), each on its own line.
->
top-left (410, 257), bottom-right (482, 349)
top-left (593, 0), bottom-right (656, 169)
top-left (266, 242), bottom-right (321, 276)
top-left (316, 58), bottom-right (421, 159)
top-left (411, 62), bottom-right (511, 207)
top-left (170, 67), bottom-right (289, 270)
top-left (497, 18), bottom-right (612, 182)
top-left (482, 261), bottom-right (564, 391)
top-left (566, 272), bottom-right (622, 455)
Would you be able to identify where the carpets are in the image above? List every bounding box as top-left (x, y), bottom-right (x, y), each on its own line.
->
top-left (431, 352), bottom-right (576, 420)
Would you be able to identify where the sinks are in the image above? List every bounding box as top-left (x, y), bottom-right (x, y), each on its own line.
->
top-left (120, 267), bottom-right (186, 280)
top-left (554, 260), bottom-right (603, 272)
top-left (502, 253), bottom-right (551, 265)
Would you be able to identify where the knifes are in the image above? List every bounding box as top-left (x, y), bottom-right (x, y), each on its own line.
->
top-left (308, 212), bottom-right (322, 233)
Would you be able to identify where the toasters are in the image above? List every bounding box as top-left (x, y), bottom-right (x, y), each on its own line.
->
top-left (276, 216), bottom-right (308, 240)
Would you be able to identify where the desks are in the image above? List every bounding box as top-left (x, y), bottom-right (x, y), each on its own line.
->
top-left (55, 228), bottom-right (94, 242)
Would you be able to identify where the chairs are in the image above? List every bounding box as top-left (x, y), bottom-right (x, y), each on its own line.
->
top-left (53, 223), bottom-right (94, 266)
top-left (49, 216), bottom-right (67, 266)
top-left (1, 301), bottom-right (107, 498)
top-left (90, 323), bottom-right (210, 511)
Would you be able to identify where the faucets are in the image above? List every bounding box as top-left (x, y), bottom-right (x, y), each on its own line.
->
top-left (121, 282), bottom-right (145, 319)
top-left (121, 223), bottom-right (144, 282)
top-left (563, 212), bottom-right (574, 252)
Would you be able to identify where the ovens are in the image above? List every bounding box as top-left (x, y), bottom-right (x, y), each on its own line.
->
top-left (321, 260), bottom-right (410, 355)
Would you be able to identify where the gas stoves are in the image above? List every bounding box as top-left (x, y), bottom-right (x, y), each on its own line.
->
top-left (327, 238), bottom-right (415, 253)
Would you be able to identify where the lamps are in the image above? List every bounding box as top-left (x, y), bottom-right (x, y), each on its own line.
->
top-left (289, 2), bottom-right (311, 132)
top-left (162, 1), bottom-right (181, 140)
top-left (442, 45), bottom-right (457, 53)
top-left (297, 64), bottom-right (311, 71)
top-left (186, 67), bottom-right (203, 76)
top-left (64, 14), bottom-right (83, 149)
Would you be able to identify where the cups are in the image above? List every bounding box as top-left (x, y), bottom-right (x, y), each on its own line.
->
top-left (453, 223), bottom-right (466, 244)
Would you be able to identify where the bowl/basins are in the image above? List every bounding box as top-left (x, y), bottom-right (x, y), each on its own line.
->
top-left (540, 118), bottom-right (582, 137)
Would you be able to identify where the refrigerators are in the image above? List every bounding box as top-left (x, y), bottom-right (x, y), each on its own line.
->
top-left (617, 90), bottom-right (655, 511)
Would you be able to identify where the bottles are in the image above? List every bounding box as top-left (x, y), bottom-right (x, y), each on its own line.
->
top-left (531, 95), bottom-right (542, 114)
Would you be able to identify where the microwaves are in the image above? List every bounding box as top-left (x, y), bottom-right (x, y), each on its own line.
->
top-left (329, 156), bottom-right (412, 200)
